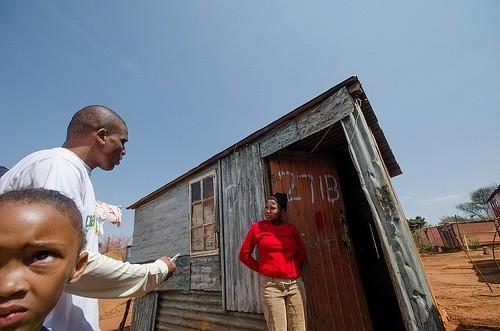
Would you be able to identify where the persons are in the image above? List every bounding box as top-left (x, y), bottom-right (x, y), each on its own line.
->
top-left (0, 188), bottom-right (89, 331)
top-left (239, 192), bottom-right (308, 331)
top-left (0, 105), bottom-right (176, 331)
